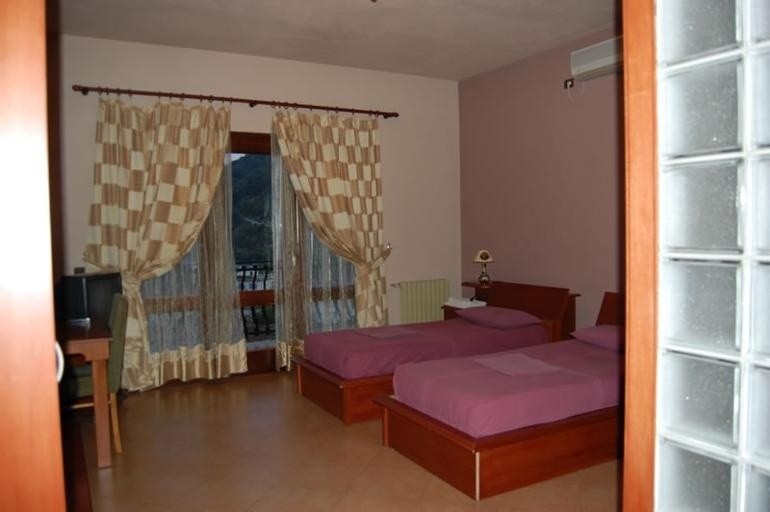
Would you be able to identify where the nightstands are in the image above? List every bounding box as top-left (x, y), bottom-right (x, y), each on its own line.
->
top-left (439, 283), bottom-right (484, 322)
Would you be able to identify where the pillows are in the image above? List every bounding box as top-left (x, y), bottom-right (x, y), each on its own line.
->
top-left (570, 324), bottom-right (625, 350)
top-left (456, 305), bottom-right (542, 329)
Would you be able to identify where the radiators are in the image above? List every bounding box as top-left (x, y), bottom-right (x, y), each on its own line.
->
top-left (399, 278), bottom-right (451, 322)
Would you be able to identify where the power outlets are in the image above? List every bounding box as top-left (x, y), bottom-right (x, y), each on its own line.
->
top-left (566, 78), bottom-right (572, 88)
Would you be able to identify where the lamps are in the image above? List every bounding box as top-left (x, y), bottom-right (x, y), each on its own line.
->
top-left (473, 248), bottom-right (494, 281)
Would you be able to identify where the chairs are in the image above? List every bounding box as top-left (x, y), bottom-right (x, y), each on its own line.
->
top-left (67, 295), bottom-right (127, 454)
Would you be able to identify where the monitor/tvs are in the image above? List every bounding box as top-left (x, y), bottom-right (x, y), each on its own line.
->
top-left (60, 271), bottom-right (123, 331)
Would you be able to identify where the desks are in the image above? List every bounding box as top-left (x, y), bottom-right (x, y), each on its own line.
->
top-left (59, 314), bottom-right (114, 469)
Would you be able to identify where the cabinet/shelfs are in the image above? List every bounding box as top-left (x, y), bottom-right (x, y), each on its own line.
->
top-left (619, 0), bottom-right (770, 512)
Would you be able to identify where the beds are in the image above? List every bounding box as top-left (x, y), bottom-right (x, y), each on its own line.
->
top-left (289, 278), bottom-right (580, 428)
top-left (373, 291), bottom-right (626, 502)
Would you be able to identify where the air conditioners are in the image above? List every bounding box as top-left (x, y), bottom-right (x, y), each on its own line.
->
top-left (568, 35), bottom-right (620, 81)
